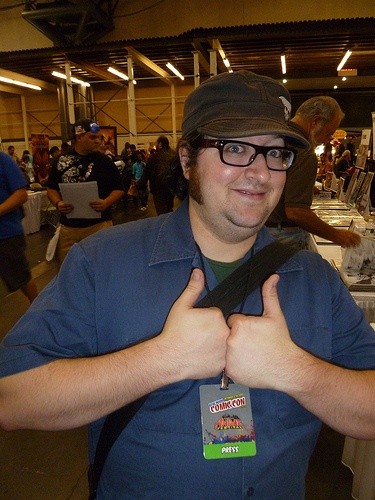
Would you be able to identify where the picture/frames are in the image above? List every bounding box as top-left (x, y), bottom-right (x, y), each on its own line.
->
top-left (96, 126), bottom-right (117, 160)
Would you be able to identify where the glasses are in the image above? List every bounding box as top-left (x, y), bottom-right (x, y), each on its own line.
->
top-left (191, 137), bottom-right (297, 172)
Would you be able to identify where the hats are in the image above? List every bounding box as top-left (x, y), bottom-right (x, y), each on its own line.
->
top-left (182, 69), bottom-right (312, 173)
top-left (71, 118), bottom-right (100, 138)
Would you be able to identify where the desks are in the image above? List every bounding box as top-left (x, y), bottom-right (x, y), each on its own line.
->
top-left (21, 191), bottom-right (42, 235)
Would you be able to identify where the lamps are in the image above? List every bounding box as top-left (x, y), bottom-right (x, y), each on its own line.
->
top-left (164, 61), bottom-right (184, 82)
top-left (50, 70), bottom-right (91, 88)
top-left (336, 48), bottom-right (353, 71)
top-left (279, 52), bottom-right (286, 77)
top-left (106, 65), bottom-right (136, 85)
top-left (217, 48), bottom-right (233, 74)
top-left (0, 74), bottom-right (40, 91)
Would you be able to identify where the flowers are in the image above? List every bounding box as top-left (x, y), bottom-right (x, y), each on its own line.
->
top-left (30, 183), bottom-right (42, 191)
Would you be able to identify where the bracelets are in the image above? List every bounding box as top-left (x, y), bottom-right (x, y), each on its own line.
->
top-left (55, 200), bottom-right (62, 209)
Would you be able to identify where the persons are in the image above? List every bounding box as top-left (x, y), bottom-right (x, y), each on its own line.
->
top-left (0, 70), bottom-right (375, 500)
